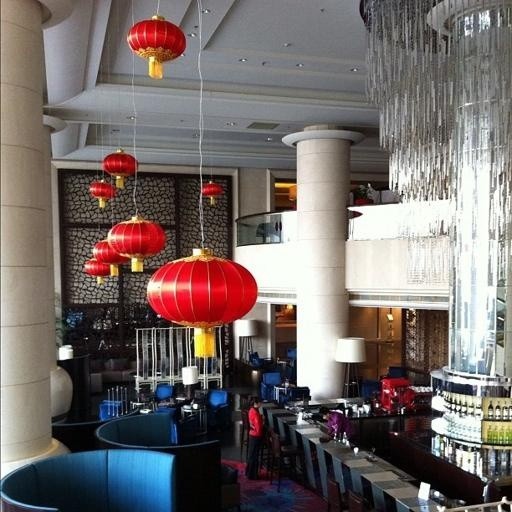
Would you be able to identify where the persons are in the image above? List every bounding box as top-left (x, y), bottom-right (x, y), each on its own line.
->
top-left (245, 396), bottom-right (264, 480)
top-left (319, 406), bottom-right (361, 446)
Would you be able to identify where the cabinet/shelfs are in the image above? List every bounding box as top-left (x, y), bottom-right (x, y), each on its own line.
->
top-left (429, 371), bottom-right (512, 487)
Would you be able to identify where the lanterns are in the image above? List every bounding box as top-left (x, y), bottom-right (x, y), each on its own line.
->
top-left (102, 148), bottom-right (138, 189)
top-left (85, 214), bottom-right (166, 286)
top-left (146, 247), bottom-right (257, 358)
top-left (201, 180), bottom-right (223, 207)
top-left (126, 15), bottom-right (187, 80)
top-left (90, 179), bottom-right (115, 209)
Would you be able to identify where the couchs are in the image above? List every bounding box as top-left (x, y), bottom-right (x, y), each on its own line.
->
top-left (1, 406), bottom-right (241, 512)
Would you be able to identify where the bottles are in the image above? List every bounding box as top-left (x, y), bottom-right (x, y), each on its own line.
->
top-left (487, 446), bottom-right (512, 466)
top-left (442, 380), bottom-right (482, 439)
top-left (486, 423), bottom-right (512, 441)
top-left (338, 432), bottom-right (350, 446)
top-left (434, 433), bottom-right (478, 469)
top-left (484, 377), bottom-right (512, 398)
top-left (488, 399), bottom-right (512, 419)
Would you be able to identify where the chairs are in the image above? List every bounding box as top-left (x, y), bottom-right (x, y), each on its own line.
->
top-left (380, 366), bottom-right (407, 379)
top-left (241, 409), bottom-right (308, 492)
top-left (346, 488), bottom-right (380, 512)
top-left (327, 479), bottom-right (349, 511)
top-left (99, 347), bottom-right (296, 433)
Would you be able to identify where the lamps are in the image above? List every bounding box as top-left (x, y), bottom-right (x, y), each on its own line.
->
top-left (335, 337), bottom-right (368, 398)
top-left (235, 319), bottom-right (260, 365)
